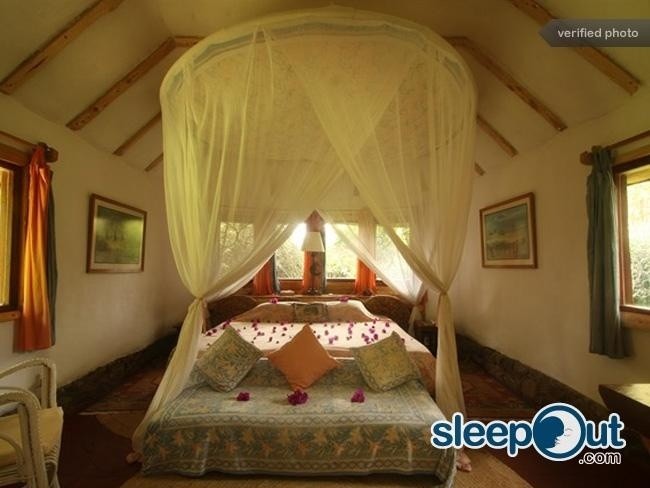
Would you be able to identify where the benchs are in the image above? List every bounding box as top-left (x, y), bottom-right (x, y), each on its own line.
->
top-left (149, 357), bottom-right (455, 484)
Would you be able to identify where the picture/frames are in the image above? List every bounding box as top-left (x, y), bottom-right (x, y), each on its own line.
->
top-left (85, 192), bottom-right (148, 274)
top-left (477, 192), bottom-right (539, 270)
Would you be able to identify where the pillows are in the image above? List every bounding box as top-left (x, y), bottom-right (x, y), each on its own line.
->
top-left (195, 322), bottom-right (423, 391)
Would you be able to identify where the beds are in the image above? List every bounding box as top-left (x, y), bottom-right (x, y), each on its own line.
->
top-left (169, 298), bottom-right (436, 402)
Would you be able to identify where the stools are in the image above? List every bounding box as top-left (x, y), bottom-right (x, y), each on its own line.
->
top-left (0, 357), bottom-right (66, 487)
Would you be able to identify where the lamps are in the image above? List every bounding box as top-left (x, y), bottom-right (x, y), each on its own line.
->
top-left (302, 230), bottom-right (325, 295)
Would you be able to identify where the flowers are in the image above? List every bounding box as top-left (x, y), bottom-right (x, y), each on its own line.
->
top-left (281, 384), bottom-right (309, 404)
top-left (224, 390), bottom-right (252, 401)
top-left (350, 387), bottom-right (367, 402)
